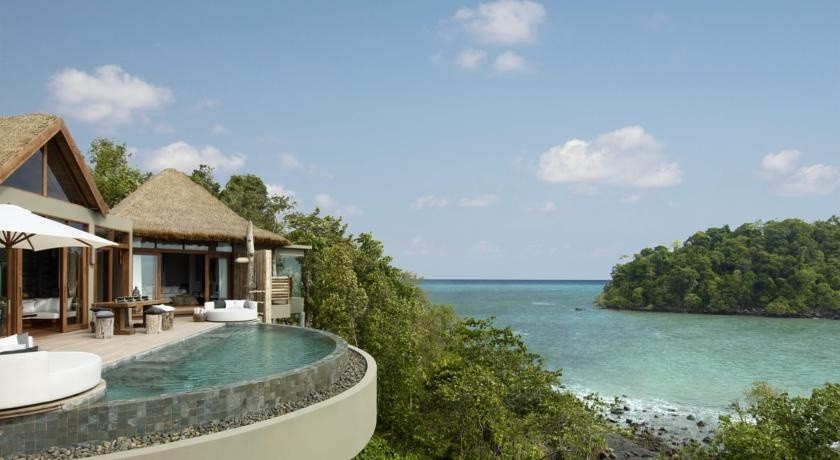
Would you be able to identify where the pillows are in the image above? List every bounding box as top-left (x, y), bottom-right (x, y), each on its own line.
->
top-left (244, 302), bottom-right (249, 308)
top-left (144, 308), bottom-right (165, 314)
top-left (96, 311), bottom-right (114, 318)
top-left (90, 308), bottom-right (110, 311)
top-left (215, 300), bottom-right (226, 308)
top-left (153, 305), bottom-right (175, 312)
top-left (17, 332), bottom-right (29, 347)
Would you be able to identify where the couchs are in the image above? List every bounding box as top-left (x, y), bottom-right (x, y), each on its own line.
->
top-left (160, 287), bottom-right (186, 298)
top-left (0, 333), bottom-right (38, 353)
top-left (22, 297), bottom-right (73, 314)
top-left (23, 296), bottom-right (77, 325)
top-left (205, 300), bottom-right (258, 322)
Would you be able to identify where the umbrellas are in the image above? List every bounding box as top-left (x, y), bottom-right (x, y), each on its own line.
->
top-left (0, 201), bottom-right (119, 336)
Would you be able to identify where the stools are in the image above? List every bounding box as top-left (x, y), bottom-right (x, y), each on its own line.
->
top-left (146, 315), bottom-right (162, 335)
top-left (96, 318), bottom-right (114, 338)
top-left (162, 312), bottom-right (175, 331)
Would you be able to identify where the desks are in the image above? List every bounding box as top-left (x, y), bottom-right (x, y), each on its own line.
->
top-left (92, 299), bottom-right (169, 336)
